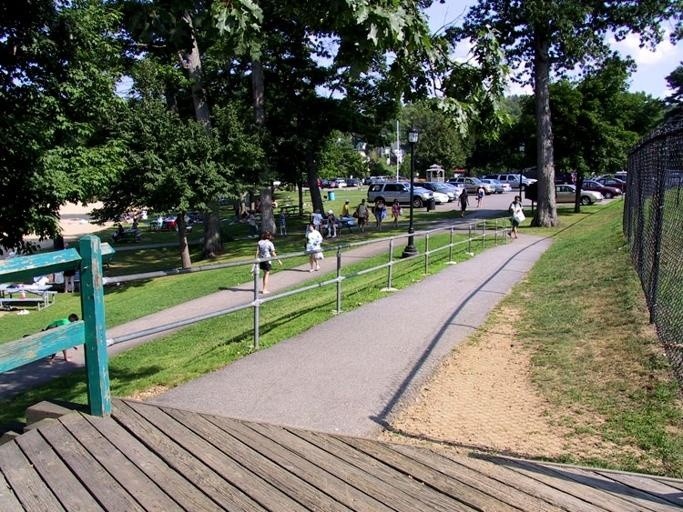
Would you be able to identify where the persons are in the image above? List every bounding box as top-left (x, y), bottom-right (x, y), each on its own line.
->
top-left (112, 216), bottom-right (192, 243)
top-left (304, 223), bottom-right (324, 272)
top-left (45, 313), bottom-right (79, 364)
top-left (458, 189), bottom-right (470, 218)
top-left (248, 199), bottom-right (401, 238)
top-left (63, 270), bottom-right (76, 293)
top-left (476, 185), bottom-right (485, 208)
top-left (255, 231), bottom-right (282, 294)
top-left (507, 196), bottom-right (524, 239)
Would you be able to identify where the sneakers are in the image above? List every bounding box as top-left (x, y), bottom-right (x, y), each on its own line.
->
top-left (308, 264), bottom-right (321, 272)
top-left (262, 290), bottom-right (271, 294)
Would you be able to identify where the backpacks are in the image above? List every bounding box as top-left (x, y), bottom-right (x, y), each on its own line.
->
top-left (478, 190), bottom-right (483, 198)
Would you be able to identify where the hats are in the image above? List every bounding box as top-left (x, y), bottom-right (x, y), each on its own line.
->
top-left (326, 209), bottom-right (334, 215)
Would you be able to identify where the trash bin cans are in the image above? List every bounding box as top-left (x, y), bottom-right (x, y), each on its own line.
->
top-left (427, 198), bottom-right (435, 210)
top-left (328, 191), bottom-right (336, 201)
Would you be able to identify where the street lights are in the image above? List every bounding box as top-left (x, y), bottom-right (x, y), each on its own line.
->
top-left (517, 138), bottom-right (526, 207)
top-left (401, 119), bottom-right (418, 260)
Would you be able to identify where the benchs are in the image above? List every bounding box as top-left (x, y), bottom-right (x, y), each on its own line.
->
top-left (278, 200), bottom-right (299, 217)
top-left (111, 212), bottom-right (178, 244)
top-left (314, 216), bottom-right (352, 237)
top-left (0, 270), bottom-right (79, 313)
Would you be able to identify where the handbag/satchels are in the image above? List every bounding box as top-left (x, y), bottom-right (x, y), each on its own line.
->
top-left (513, 208), bottom-right (526, 224)
top-left (352, 205), bottom-right (360, 219)
top-left (380, 208), bottom-right (387, 219)
top-left (399, 207), bottom-right (404, 216)
top-left (304, 242), bottom-right (307, 251)
